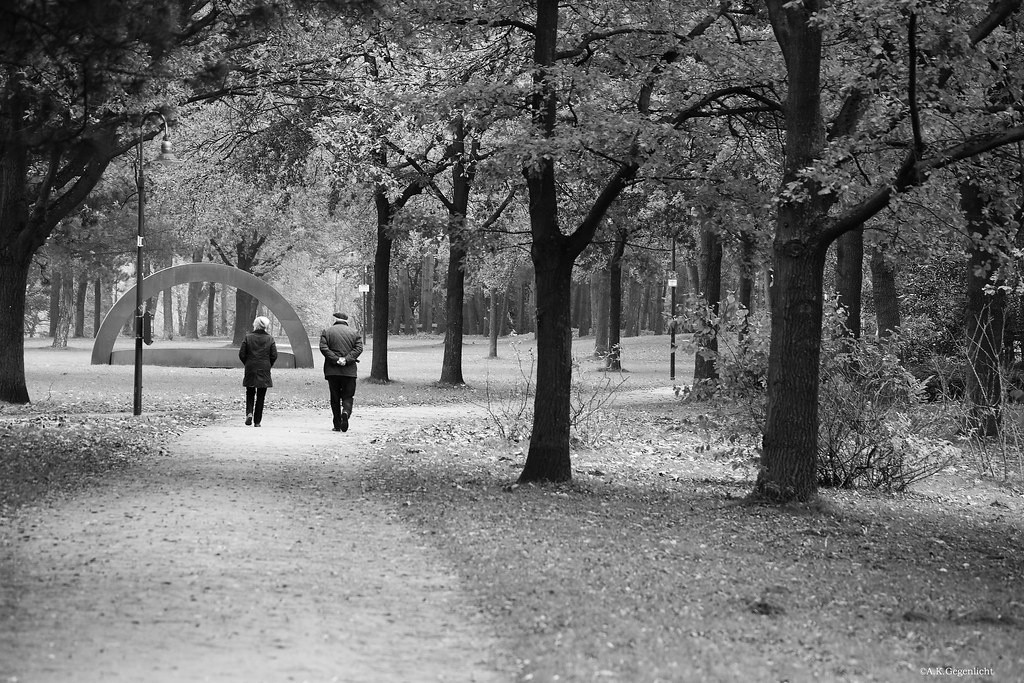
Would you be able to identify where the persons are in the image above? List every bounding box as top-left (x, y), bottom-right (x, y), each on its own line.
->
top-left (238, 316), bottom-right (278, 427)
top-left (319, 312), bottom-right (363, 431)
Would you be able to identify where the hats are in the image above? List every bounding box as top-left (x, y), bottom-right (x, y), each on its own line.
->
top-left (333, 312), bottom-right (348, 320)
top-left (254, 316), bottom-right (269, 329)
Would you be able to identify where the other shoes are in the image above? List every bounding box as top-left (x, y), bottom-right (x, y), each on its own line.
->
top-left (332, 428), bottom-right (340, 431)
top-left (254, 424), bottom-right (261, 427)
top-left (245, 413), bottom-right (252, 425)
top-left (341, 412), bottom-right (348, 432)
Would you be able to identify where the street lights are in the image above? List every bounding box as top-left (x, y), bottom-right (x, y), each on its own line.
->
top-left (133, 111), bottom-right (171, 415)
